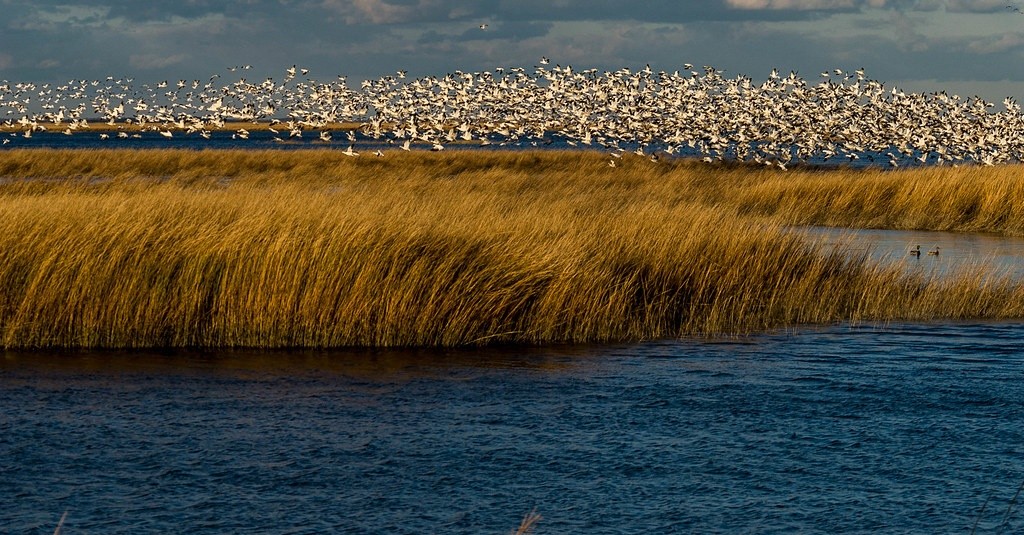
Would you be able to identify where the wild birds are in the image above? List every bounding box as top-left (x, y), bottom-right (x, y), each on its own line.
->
top-left (479, 24), bottom-right (488, 29)
top-left (927, 246), bottom-right (940, 254)
top-left (911, 245), bottom-right (921, 254)
top-left (0, 56), bottom-right (1024, 171)
